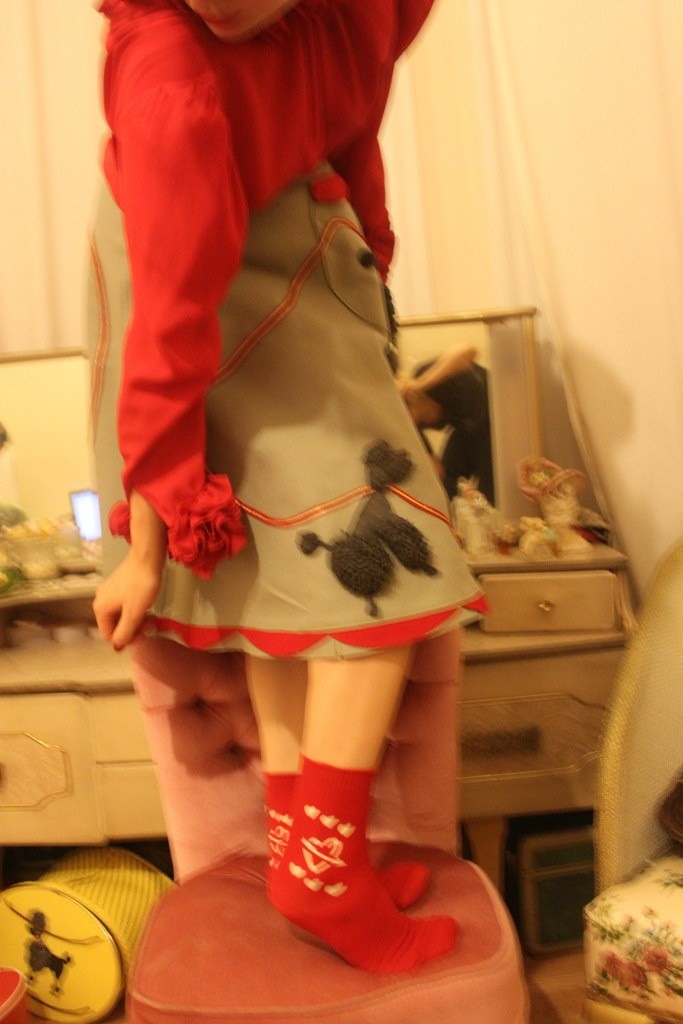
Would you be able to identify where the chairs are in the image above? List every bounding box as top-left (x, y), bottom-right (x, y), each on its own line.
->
top-left (122, 624), bottom-right (532, 1024)
top-left (580, 533), bottom-right (683, 1024)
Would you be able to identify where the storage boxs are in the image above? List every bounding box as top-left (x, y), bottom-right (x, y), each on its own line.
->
top-left (517, 828), bottom-right (598, 945)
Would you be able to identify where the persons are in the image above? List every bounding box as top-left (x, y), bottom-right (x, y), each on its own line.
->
top-left (79, 2), bottom-right (491, 975)
top-left (400, 346), bottom-right (492, 508)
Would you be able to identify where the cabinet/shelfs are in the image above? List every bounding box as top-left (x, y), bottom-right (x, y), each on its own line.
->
top-left (0, 522), bottom-right (636, 955)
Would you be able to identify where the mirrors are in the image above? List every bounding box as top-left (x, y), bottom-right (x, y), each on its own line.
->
top-left (0, 308), bottom-right (544, 550)
